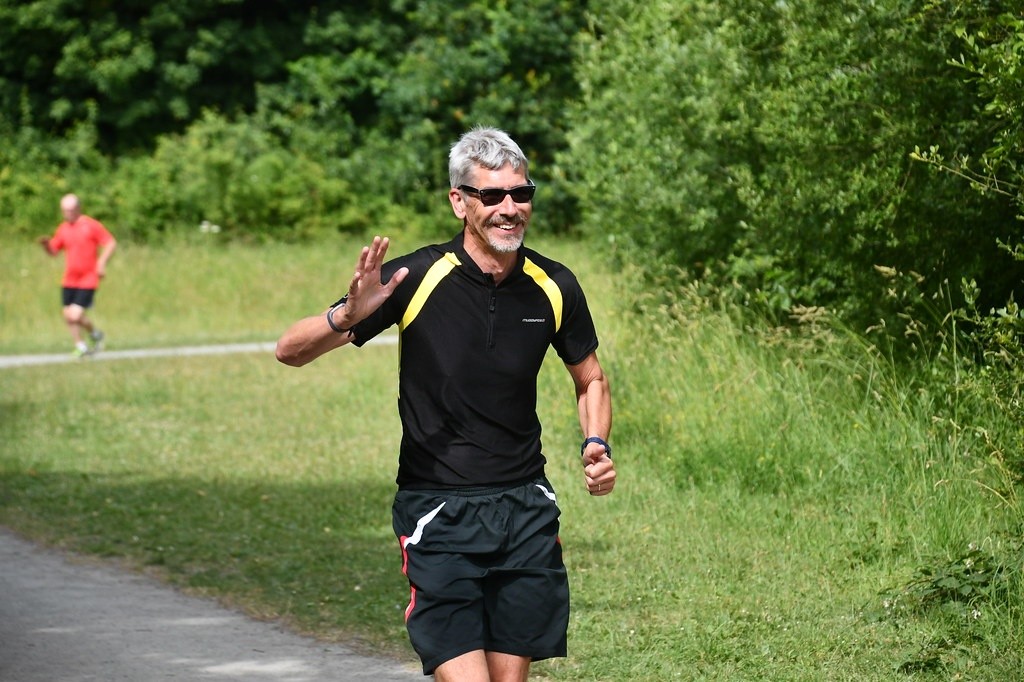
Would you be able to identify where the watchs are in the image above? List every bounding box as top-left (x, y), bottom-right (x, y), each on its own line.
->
top-left (581, 436), bottom-right (612, 459)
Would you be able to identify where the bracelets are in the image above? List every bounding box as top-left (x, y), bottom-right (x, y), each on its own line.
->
top-left (327, 304), bottom-right (355, 338)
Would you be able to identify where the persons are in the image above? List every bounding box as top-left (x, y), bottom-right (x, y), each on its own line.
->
top-left (276, 123), bottom-right (616, 682)
top-left (37, 193), bottom-right (117, 359)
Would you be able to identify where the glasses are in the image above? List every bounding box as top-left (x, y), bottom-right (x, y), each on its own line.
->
top-left (456, 179), bottom-right (536, 206)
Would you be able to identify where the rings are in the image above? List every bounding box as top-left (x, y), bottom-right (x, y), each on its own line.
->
top-left (598, 484), bottom-right (601, 491)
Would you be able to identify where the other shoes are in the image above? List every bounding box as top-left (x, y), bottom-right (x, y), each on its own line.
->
top-left (74, 349), bottom-right (90, 359)
top-left (92, 331), bottom-right (105, 353)
